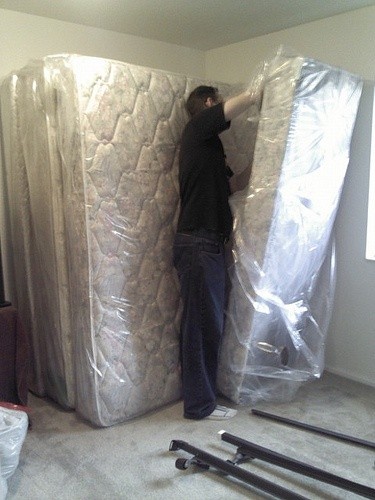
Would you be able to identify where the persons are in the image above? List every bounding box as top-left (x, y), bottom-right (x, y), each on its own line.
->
top-left (173, 79), bottom-right (254, 421)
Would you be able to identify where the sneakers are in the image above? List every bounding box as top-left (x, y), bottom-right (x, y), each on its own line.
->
top-left (203, 405), bottom-right (238, 420)
